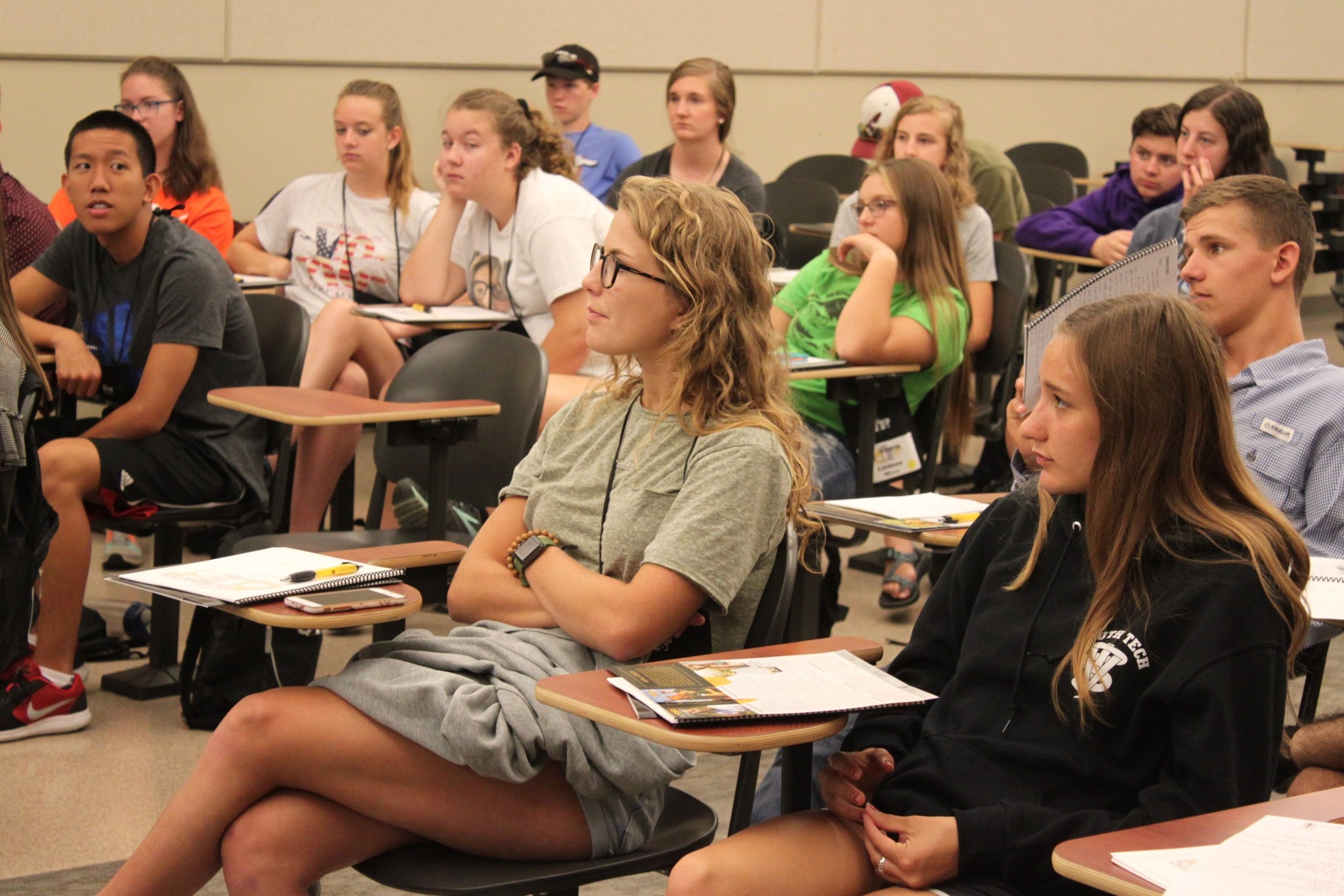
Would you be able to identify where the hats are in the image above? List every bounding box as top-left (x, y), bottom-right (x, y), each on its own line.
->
top-left (851, 82), bottom-right (923, 160)
top-left (531, 45), bottom-right (599, 85)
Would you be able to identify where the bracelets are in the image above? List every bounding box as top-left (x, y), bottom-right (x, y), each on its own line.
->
top-left (506, 528), bottom-right (559, 578)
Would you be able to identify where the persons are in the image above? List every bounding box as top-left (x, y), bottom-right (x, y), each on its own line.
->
top-left (96, 175), bottom-right (830, 896)
top-left (0, 166), bottom-right (77, 417)
top-left (378, 88), bottom-right (642, 537)
top-left (0, 107), bottom-right (269, 745)
top-left (51, 57), bottom-right (238, 260)
top-left (665, 294), bottom-right (1312, 896)
top-left (225, 77), bottom-right (441, 537)
top-left (529, 37), bottom-right (1344, 611)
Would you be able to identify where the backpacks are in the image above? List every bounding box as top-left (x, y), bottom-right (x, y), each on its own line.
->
top-left (179, 603), bottom-right (323, 732)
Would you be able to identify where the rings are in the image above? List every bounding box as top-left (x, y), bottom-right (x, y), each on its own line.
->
top-left (878, 856), bottom-right (885, 874)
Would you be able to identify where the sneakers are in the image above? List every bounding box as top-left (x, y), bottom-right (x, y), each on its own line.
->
top-left (1, 639), bottom-right (90, 682)
top-left (391, 477), bottom-right (482, 538)
top-left (0, 659), bottom-right (91, 742)
top-left (103, 529), bottom-right (142, 570)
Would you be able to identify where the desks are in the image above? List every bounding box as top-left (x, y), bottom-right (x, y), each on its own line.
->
top-left (1014, 244), bottom-right (1107, 269)
top-left (1052, 781), bottom-right (1344, 896)
top-left (822, 492), bottom-right (1008, 548)
top-left (784, 219), bottom-right (839, 238)
top-left (206, 541), bottom-right (468, 635)
top-left (1069, 174), bottom-right (1114, 194)
top-left (348, 303), bottom-right (493, 331)
top-left (778, 359), bottom-right (922, 381)
top-left (534, 636), bottom-right (883, 754)
top-left (206, 384), bottom-right (501, 427)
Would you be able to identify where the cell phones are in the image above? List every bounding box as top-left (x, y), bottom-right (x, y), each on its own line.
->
top-left (284, 588), bottom-right (407, 614)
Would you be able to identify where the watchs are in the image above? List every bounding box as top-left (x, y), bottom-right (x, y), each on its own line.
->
top-left (512, 534), bottom-right (558, 586)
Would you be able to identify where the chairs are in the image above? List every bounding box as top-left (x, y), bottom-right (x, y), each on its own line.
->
top-left (0, 138), bottom-right (1344, 896)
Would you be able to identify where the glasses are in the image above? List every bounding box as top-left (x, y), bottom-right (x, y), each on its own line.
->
top-left (115, 98), bottom-right (179, 117)
top-left (851, 199), bottom-right (897, 222)
top-left (588, 244), bottom-right (667, 288)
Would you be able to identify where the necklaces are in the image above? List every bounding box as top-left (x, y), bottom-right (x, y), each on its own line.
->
top-left (667, 143), bottom-right (725, 186)
top-left (572, 120), bottom-right (592, 165)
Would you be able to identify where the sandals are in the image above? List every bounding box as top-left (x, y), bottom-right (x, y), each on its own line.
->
top-left (878, 549), bottom-right (927, 609)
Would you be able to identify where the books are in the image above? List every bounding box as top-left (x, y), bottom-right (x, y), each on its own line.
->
top-left (1287, 555), bottom-right (1344, 620)
top-left (1111, 813), bottom-right (1344, 896)
top-left (606, 649), bottom-right (949, 737)
top-left (776, 352), bottom-right (847, 370)
top-left (104, 544), bottom-right (410, 606)
top-left (354, 300), bottom-right (520, 325)
top-left (232, 273), bottom-right (292, 287)
top-left (807, 492), bottom-right (991, 533)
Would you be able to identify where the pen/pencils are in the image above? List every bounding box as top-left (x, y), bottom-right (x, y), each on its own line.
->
top-left (412, 303), bottom-right (432, 312)
top-left (235, 278), bottom-right (242, 283)
top-left (938, 511), bottom-right (983, 524)
top-left (281, 562), bottom-right (362, 583)
